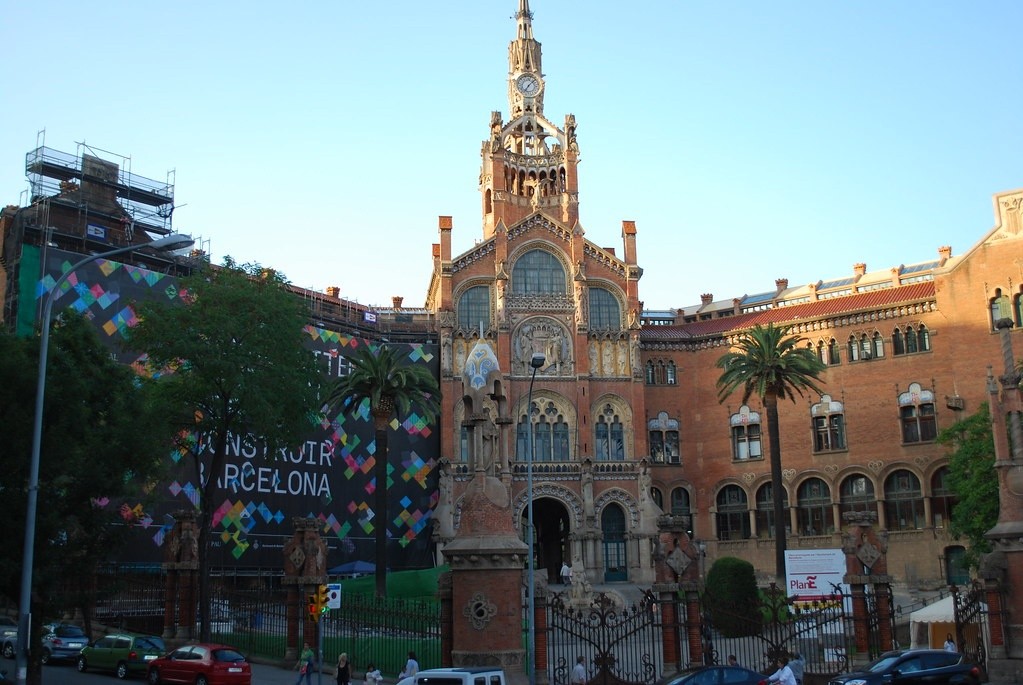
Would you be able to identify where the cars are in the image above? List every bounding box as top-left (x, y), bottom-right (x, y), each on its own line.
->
top-left (74, 631), bottom-right (169, 679)
top-left (650, 665), bottom-right (771, 685)
top-left (0, 615), bottom-right (18, 654)
top-left (145, 642), bottom-right (253, 685)
top-left (1, 621), bottom-right (89, 666)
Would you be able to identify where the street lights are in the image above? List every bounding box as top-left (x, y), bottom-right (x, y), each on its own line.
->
top-left (12, 232), bottom-right (197, 685)
top-left (525, 350), bottom-right (548, 685)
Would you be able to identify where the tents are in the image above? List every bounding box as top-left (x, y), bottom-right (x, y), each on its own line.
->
top-left (909, 593), bottom-right (988, 655)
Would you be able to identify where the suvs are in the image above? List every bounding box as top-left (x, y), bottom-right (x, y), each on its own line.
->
top-left (826, 647), bottom-right (982, 685)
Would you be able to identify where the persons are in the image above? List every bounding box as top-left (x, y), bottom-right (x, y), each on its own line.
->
top-left (570, 656), bottom-right (585, 685)
top-left (363, 663), bottom-right (383, 685)
top-left (292, 641), bottom-right (314, 685)
top-left (944, 633), bottom-right (956, 651)
top-left (398, 652), bottom-right (419, 682)
top-left (336, 652), bottom-right (351, 685)
top-left (728, 655), bottom-right (739, 667)
top-left (788, 652), bottom-right (806, 685)
top-left (560, 562), bottom-right (569, 587)
top-left (764, 657), bottom-right (797, 685)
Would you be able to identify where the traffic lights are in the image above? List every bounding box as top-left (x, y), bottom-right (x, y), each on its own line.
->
top-left (317, 584), bottom-right (330, 615)
top-left (307, 593), bottom-right (319, 623)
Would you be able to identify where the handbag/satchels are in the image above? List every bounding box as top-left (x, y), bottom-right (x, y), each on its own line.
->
top-left (333, 664), bottom-right (339, 679)
top-left (410, 663), bottom-right (417, 676)
top-left (301, 661), bottom-right (308, 675)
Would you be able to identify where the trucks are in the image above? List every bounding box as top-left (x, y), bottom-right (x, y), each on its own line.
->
top-left (395, 665), bottom-right (506, 685)
